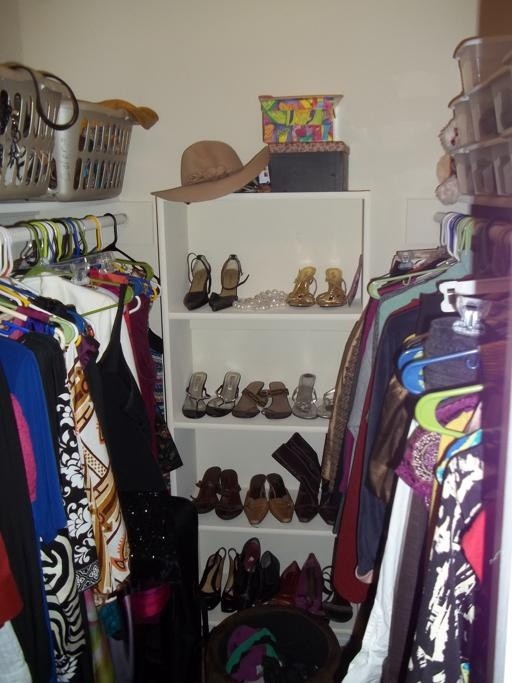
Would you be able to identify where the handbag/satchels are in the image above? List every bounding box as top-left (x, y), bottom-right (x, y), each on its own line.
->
top-left (119, 495), bottom-right (197, 584)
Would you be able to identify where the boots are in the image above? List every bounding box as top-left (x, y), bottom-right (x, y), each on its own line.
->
top-left (271, 431), bottom-right (336, 524)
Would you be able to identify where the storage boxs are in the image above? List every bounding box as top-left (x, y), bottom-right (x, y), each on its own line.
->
top-left (268, 140), bottom-right (351, 192)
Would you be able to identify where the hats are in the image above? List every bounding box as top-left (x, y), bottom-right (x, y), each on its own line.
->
top-left (150, 140), bottom-right (269, 202)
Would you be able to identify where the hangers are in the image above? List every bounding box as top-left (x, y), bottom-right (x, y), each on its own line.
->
top-left (366, 211), bottom-right (512, 438)
top-left (0, 212), bottom-right (159, 345)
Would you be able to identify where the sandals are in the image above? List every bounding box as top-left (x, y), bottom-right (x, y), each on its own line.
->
top-left (191, 466), bottom-right (293, 525)
top-left (209, 253), bottom-right (249, 311)
top-left (199, 537), bottom-right (353, 623)
top-left (286, 267), bottom-right (317, 307)
top-left (317, 269), bottom-right (347, 308)
top-left (183, 253), bottom-right (211, 310)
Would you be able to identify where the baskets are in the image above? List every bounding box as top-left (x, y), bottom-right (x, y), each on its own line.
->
top-left (1, 67), bottom-right (133, 202)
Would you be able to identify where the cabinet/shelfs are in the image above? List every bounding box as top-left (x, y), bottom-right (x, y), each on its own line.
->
top-left (157, 188), bottom-right (371, 647)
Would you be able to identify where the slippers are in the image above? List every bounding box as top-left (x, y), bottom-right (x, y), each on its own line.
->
top-left (182, 373), bottom-right (336, 420)
